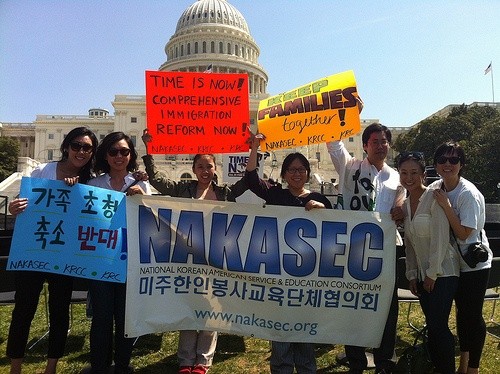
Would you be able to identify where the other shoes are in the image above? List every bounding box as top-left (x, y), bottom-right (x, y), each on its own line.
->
top-left (177, 365), bottom-right (209, 374)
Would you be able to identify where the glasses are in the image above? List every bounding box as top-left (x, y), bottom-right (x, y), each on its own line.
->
top-left (368, 141), bottom-right (391, 146)
top-left (70, 142), bottom-right (94, 152)
top-left (437, 156), bottom-right (461, 165)
top-left (287, 168), bottom-right (307, 173)
top-left (397, 152), bottom-right (425, 172)
top-left (108, 148), bottom-right (131, 157)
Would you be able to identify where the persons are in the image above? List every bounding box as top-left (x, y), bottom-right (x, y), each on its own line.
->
top-left (65, 132), bottom-right (152, 374)
top-left (247, 132), bottom-right (334, 374)
top-left (427, 142), bottom-right (492, 373)
top-left (6, 127), bottom-right (99, 374)
top-left (395, 150), bottom-right (457, 374)
top-left (326, 123), bottom-right (406, 374)
top-left (142, 129), bottom-right (257, 374)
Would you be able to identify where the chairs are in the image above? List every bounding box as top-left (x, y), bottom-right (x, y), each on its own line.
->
top-left (0, 237), bottom-right (87, 350)
top-left (484, 257), bottom-right (500, 321)
top-left (398, 257), bottom-right (428, 338)
